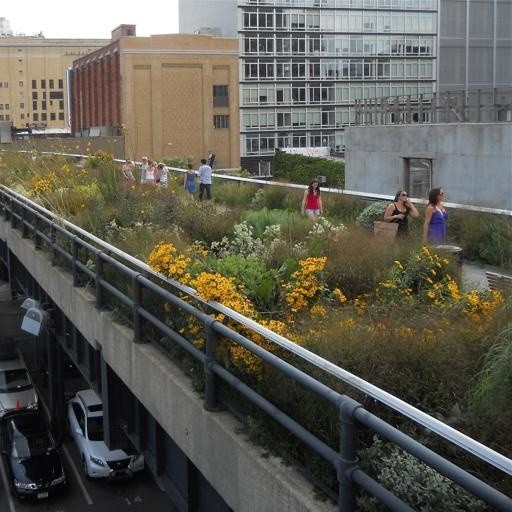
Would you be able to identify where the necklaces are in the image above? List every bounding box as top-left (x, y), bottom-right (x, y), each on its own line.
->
top-left (397, 205), bottom-right (404, 213)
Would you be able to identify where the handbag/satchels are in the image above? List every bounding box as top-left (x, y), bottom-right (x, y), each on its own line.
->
top-left (374, 221), bottom-right (399, 243)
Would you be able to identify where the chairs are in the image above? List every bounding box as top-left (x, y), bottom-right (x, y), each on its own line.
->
top-left (485, 270), bottom-right (512, 292)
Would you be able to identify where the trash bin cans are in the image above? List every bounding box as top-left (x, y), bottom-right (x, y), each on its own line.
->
top-left (430, 244), bottom-right (463, 287)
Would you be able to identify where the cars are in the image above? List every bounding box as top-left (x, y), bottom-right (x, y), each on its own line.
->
top-left (0, 345), bottom-right (145, 503)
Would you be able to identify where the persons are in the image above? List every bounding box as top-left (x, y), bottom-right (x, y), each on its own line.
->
top-left (420, 187), bottom-right (450, 247)
top-left (383, 191), bottom-right (420, 240)
top-left (301, 178), bottom-right (324, 222)
top-left (122, 157), bottom-right (213, 202)
